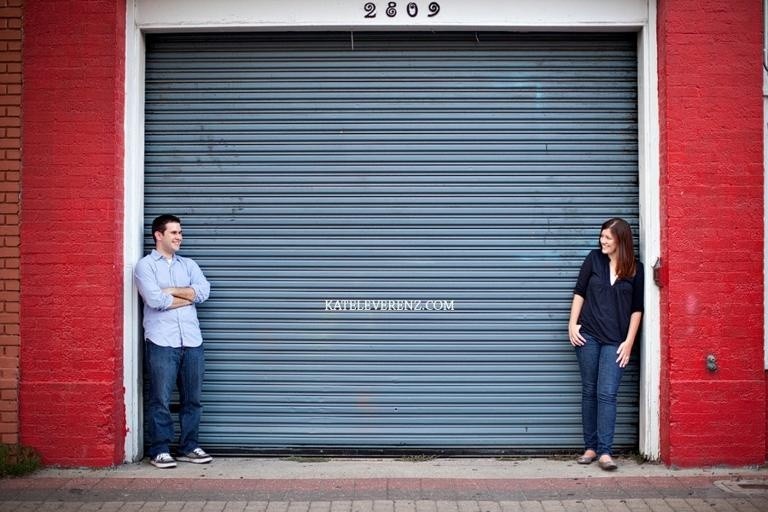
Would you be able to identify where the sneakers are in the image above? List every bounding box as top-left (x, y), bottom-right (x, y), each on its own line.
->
top-left (597, 453), bottom-right (617, 469)
top-left (175, 447), bottom-right (213, 463)
top-left (148, 451), bottom-right (178, 468)
top-left (577, 455), bottom-right (597, 464)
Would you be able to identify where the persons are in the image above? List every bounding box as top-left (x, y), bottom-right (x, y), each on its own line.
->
top-left (135, 216), bottom-right (214, 468)
top-left (568, 216), bottom-right (643, 469)
